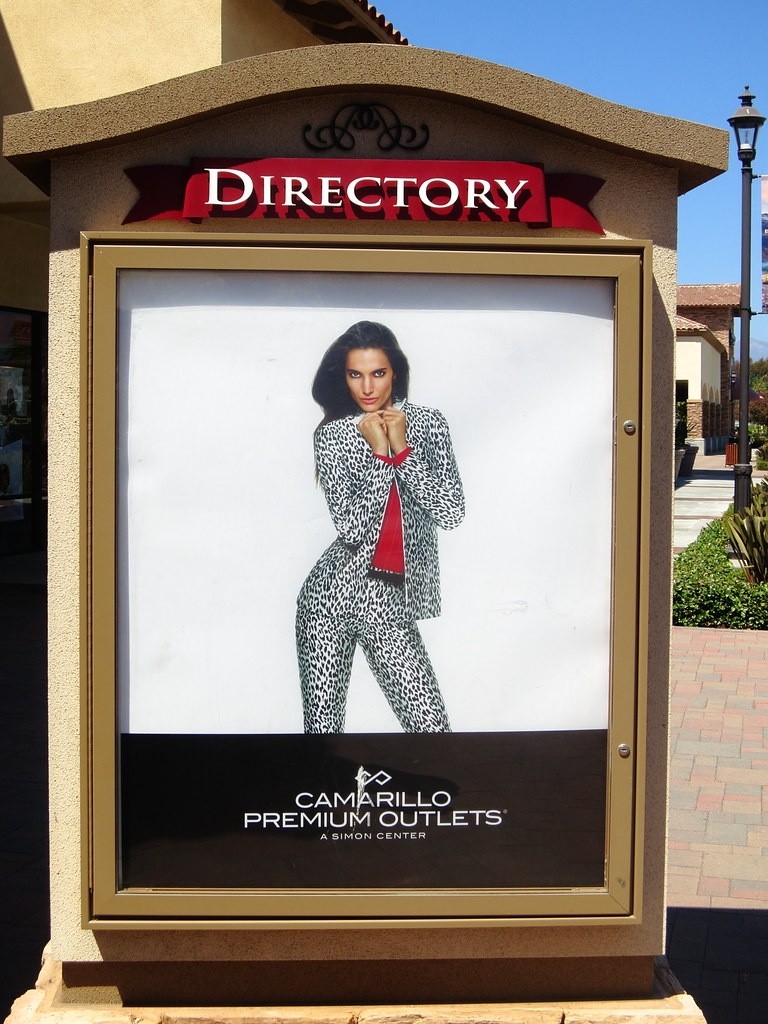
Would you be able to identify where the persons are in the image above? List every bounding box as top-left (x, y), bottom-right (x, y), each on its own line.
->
top-left (296, 321), bottom-right (465, 734)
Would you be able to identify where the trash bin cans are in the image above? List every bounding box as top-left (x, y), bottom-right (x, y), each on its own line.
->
top-left (725, 443), bottom-right (738, 467)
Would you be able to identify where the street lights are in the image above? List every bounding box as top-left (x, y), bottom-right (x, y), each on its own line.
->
top-left (725, 84), bottom-right (767, 558)
